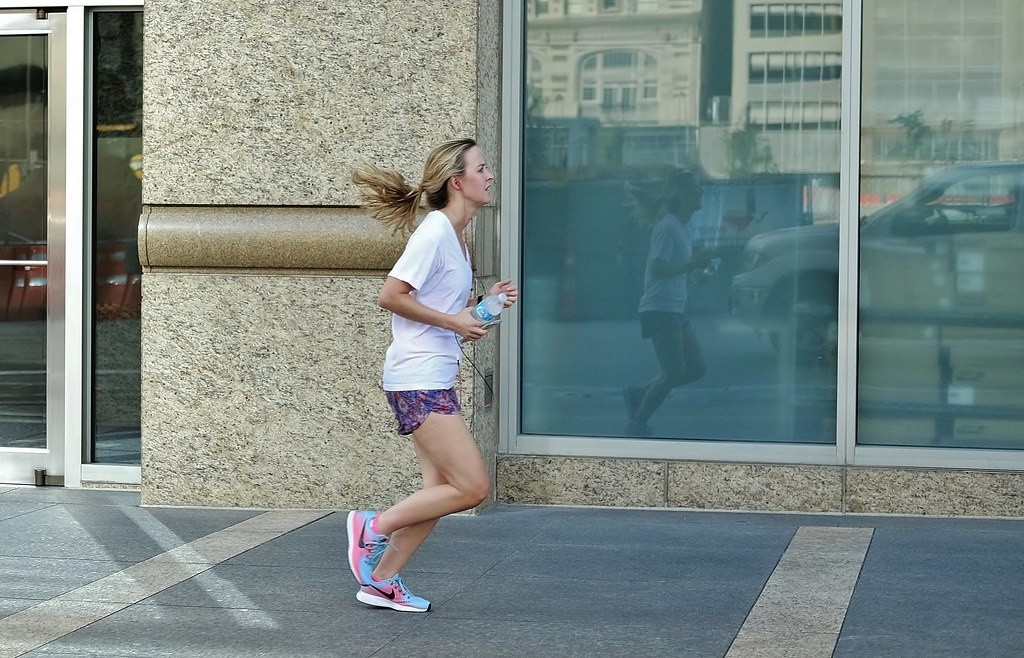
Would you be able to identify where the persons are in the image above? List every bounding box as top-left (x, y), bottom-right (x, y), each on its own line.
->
top-left (621, 166), bottom-right (730, 437)
top-left (347, 138), bottom-right (518, 613)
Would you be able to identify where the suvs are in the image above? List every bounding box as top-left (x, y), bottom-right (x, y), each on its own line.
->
top-left (732, 160), bottom-right (1023, 366)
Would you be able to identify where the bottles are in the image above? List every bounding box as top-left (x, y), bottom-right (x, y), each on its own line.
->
top-left (470, 293), bottom-right (507, 323)
top-left (704, 258), bottom-right (721, 274)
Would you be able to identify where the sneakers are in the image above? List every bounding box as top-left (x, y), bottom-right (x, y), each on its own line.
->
top-left (622, 385), bottom-right (643, 424)
top-left (625, 420), bottom-right (652, 437)
top-left (346, 510), bottom-right (400, 588)
top-left (355, 573), bottom-right (432, 613)
